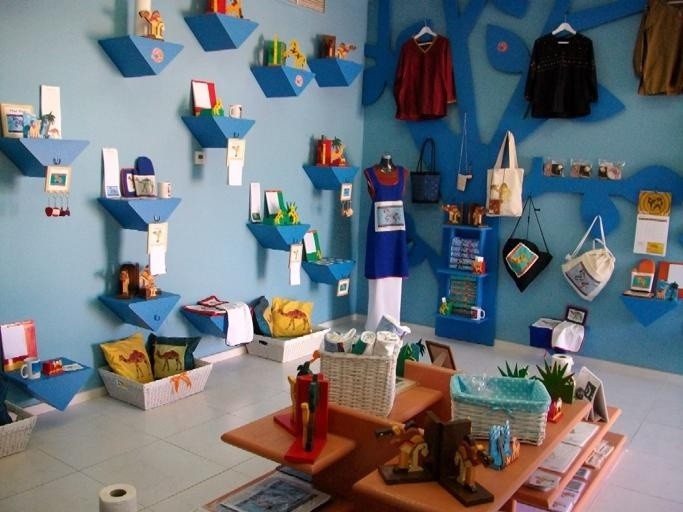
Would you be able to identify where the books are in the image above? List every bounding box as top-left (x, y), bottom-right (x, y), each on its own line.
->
top-left (218, 470), bottom-right (332, 512)
top-left (525, 419), bottom-right (614, 512)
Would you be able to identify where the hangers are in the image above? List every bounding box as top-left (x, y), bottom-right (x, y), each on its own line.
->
top-left (552, 11), bottom-right (577, 44)
top-left (414, 14), bottom-right (437, 45)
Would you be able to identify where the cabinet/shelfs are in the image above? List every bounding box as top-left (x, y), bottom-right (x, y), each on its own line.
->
top-left (207, 357), bottom-right (629, 512)
top-left (433, 201), bottom-right (501, 346)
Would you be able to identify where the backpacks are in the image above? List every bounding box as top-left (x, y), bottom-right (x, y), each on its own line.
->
top-left (502, 237), bottom-right (553, 293)
top-left (560, 248), bottom-right (617, 305)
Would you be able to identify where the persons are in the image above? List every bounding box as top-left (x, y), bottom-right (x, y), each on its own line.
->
top-left (364, 153), bottom-right (410, 334)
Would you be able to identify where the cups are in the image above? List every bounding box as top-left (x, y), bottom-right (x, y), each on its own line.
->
top-left (228, 103), bottom-right (244, 119)
top-left (20, 357), bottom-right (41, 380)
top-left (157, 181), bottom-right (175, 199)
top-left (470, 306), bottom-right (486, 321)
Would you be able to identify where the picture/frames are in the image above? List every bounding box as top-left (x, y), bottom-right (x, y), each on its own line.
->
top-left (122, 168), bottom-right (138, 198)
top-left (425, 339), bottom-right (457, 371)
top-left (336, 279), bottom-right (350, 296)
top-left (338, 183), bottom-right (353, 203)
top-left (44, 166), bottom-right (70, 193)
top-left (0, 102), bottom-right (37, 138)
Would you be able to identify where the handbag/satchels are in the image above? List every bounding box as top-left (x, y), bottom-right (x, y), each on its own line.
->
top-left (484, 163), bottom-right (527, 219)
top-left (409, 169), bottom-right (442, 206)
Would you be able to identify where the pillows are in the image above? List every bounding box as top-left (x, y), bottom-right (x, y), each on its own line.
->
top-left (99, 330), bottom-right (154, 387)
top-left (146, 333), bottom-right (202, 380)
top-left (271, 297), bottom-right (314, 337)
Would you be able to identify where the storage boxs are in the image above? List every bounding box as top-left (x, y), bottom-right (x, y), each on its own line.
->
top-left (529, 316), bottom-right (567, 356)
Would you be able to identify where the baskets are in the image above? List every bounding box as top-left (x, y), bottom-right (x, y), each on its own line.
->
top-left (246, 324), bottom-right (331, 362)
top-left (316, 338), bottom-right (398, 418)
top-left (449, 373), bottom-right (552, 447)
top-left (98, 353), bottom-right (211, 412)
